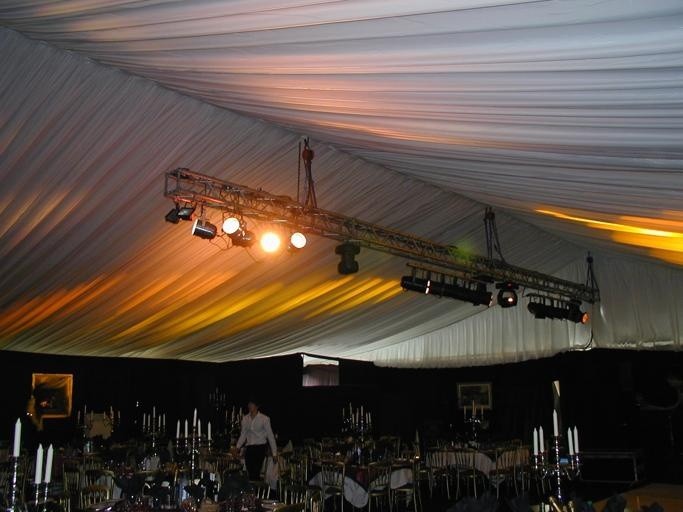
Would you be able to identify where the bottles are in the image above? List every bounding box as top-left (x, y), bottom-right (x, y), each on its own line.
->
top-left (78, 450), bottom-right (244, 512)
top-left (538, 495), bottom-right (598, 512)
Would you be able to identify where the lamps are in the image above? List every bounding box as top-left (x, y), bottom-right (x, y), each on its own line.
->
top-left (161, 134), bottom-right (600, 328)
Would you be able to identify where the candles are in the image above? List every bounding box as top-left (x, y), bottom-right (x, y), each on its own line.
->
top-left (566, 426), bottom-right (574, 456)
top-left (551, 408), bottom-right (559, 437)
top-left (573, 424), bottom-right (580, 454)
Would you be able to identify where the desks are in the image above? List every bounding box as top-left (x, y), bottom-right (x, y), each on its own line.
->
top-left (592, 482), bottom-right (682, 511)
top-left (575, 449), bottom-right (646, 483)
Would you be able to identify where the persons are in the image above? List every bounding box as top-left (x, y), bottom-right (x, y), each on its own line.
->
top-left (232, 399), bottom-right (280, 483)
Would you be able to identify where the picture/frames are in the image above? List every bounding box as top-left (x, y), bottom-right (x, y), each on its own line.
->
top-left (31, 372), bottom-right (74, 418)
top-left (456, 380), bottom-right (492, 408)
